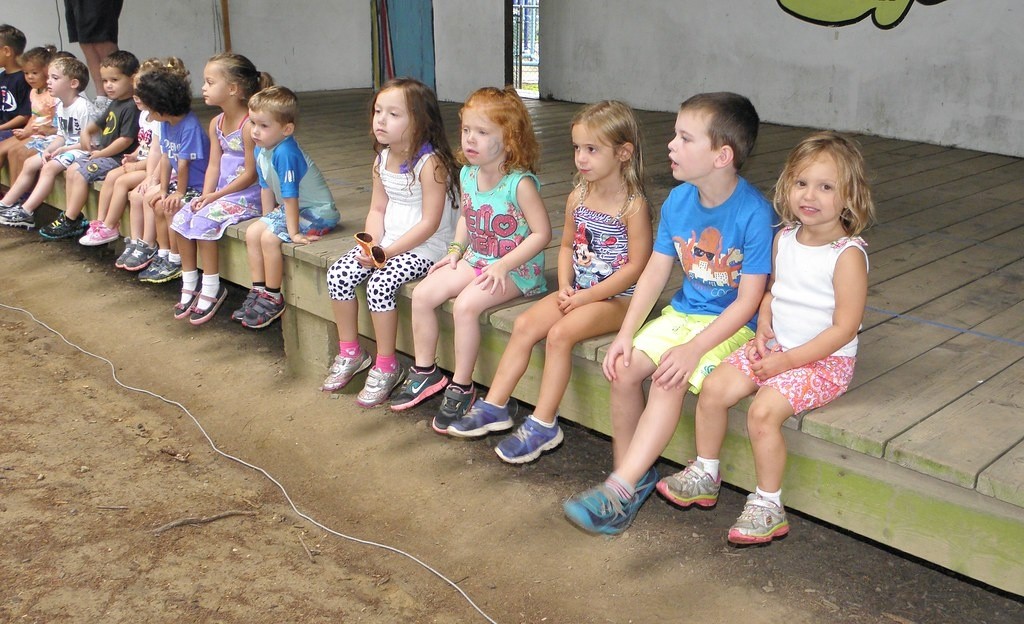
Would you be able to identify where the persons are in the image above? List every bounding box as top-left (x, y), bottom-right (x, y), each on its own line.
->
top-left (390, 87), bottom-right (552, 433)
top-left (137, 69), bottom-right (211, 283)
top-left (0, 57), bottom-right (100, 230)
top-left (232, 85), bottom-right (341, 328)
top-left (0, 45), bottom-right (57, 189)
top-left (39, 50), bottom-right (140, 239)
top-left (323, 78), bottom-right (461, 408)
top-left (563, 92), bottom-right (779, 536)
top-left (655, 130), bottom-right (873, 544)
top-left (170, 52), bottom-right (274, 324)
top-left (114, 58), bottom-right (185, 270)
top-left (447, 99), bottom-right (653, 463)
top-left (78, 76), bottom-right (155, 246)
top-left (64, 0), bottom-right (124, 108)
top-left (0, 24), bottom-right (32, 143)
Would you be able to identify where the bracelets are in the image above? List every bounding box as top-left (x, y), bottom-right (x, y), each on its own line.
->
top-left (448, 242), bottom-right (465, 259)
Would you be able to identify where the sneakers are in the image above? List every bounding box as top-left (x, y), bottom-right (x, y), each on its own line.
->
top-left (123, 241), bottom-right (159, 271)
top-left (242, 291), bottom-right (285, 329)
top-left (146, 257), bottom-right (188, 284)
top-left (495, 412), bottom-right (564, 464)
top-left (323, 349), bottom-right (372, 391)
top-left (38, 209), bottom-right (85, 239)
top-left (356, 361), bottom-right (406, 407)
top-left (728, 491), bottom-right (789, 545)
top-left (564, 467), bottom-right (659, 535)
top-left (0, 203), bottom-right (35, 230)
top-left (0, 198), bottom-right (22, 212)
top-left (390, 363), bottom-right (448, 411)
top-left (114, 237), bottom-right (141, 268)
top-left (232, 289), bottom-right (264, 320)
top-left (138, 251), bottom-right (168, 281)
top-left (432, 379), bottom-right (478, 433)
top-left (445, 394), bottom-right (514, 438)
top-left (656, 458), bottom-right (721, 508)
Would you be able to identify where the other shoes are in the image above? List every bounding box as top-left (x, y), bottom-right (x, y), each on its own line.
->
top-left (190, 286), bottom-right (228, 325)
top-left (78, 217), bottom-right (119, 246)
top-left (173, 281), bottom-right (203, 320)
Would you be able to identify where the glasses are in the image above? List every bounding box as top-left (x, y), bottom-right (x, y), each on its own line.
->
top-left (354, 230), bottom-right (385, 269)
